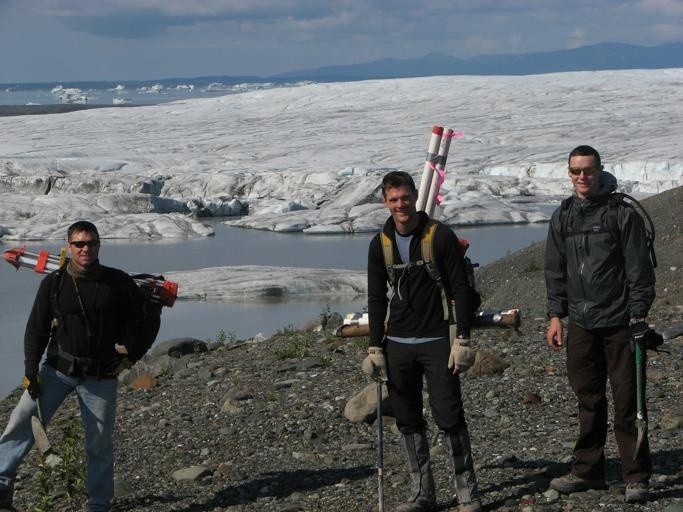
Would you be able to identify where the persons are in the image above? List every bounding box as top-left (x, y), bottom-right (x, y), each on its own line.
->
top-left (361, 170), bottom-right (484, 511)
top-left (0, 220), bottom-right (161, 512)
top-left (544, 145), bottom-right (655, 504)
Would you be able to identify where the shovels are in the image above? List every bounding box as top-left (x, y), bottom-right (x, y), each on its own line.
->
top-left (633, 339), bottom-right (647, 460)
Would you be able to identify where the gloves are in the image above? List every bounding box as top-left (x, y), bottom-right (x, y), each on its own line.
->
top-left (448, 339), bottom-right (475, 374)
top-left (630, 322), bottom-right (663, 349)
top-left (23, 375), bottom-right (39, 401)
top-left (362, 346), bottom-right (388, 382)
top-left (105, 357), bottom-right (133, 377)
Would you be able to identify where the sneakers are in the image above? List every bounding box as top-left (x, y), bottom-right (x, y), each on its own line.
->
top-left (460, 499), bottom-right (483, 512)
top-left (551, 474), bottom-right (605, 494)
top-left (625, 481), bottom-right (649, 502)
top-left (394, 501), bottom-right (436, 512)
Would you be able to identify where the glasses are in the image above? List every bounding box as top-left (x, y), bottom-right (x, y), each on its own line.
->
top-left (569, 167), bottom-right (597, 175)
top-left (71, 240), bottom-right (98, 248)
